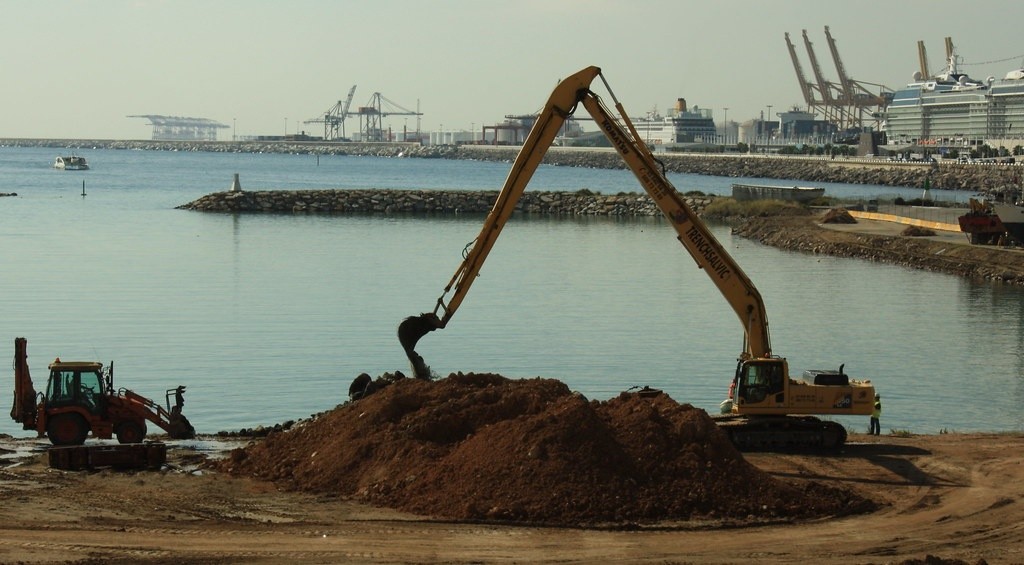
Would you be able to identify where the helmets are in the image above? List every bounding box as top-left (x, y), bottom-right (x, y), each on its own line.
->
top-left (875, 393), bottom-right (880, 398)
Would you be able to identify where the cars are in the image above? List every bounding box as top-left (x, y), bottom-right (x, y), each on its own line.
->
top-left (888, 151), bottom-right (1016, 164)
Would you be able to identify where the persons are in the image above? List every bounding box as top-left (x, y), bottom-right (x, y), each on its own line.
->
top-left (70, 374), bottom-right (94, 407)
top-left (758, 369), bottom-right (775, 396)
top-left (867, 394), bottom-right (882, 437)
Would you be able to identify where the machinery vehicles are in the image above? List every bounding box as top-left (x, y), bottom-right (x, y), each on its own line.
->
top-left (10, 337), bottom-right (195, 445)
top-left (396, 66), bottom-right (875, 456)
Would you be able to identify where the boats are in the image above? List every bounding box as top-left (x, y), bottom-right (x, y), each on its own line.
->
top-left (55, 156), bottom-right (89, 170)
